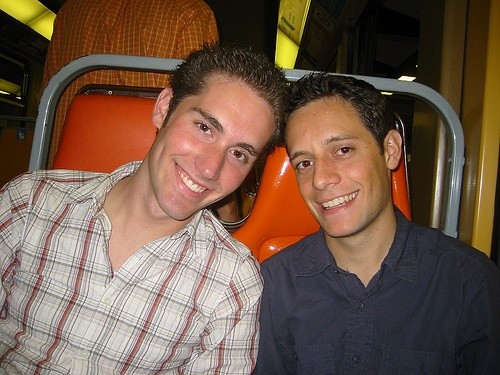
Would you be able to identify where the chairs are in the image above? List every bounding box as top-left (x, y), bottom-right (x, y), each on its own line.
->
top-left (46, 54), bottom-right (234, 243)
top-left (0, 126), bottom-right (33, 183)
top-left (229, 139), bottom-right (414, 267)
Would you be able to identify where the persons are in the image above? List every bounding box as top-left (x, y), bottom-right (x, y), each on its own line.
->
top-left (0, 41), bottom-right (294, 375)
top-left (259, 70), bottom-right (500, 375)
top-left (38, 0), bottom-right (220, 171)
